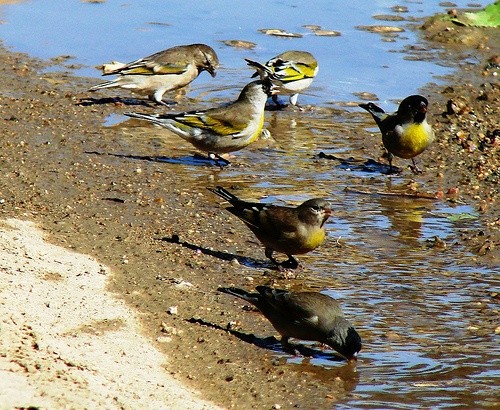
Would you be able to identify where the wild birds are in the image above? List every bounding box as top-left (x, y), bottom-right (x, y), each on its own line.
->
top-left (215, 283), bottom-right (364, 368)
top-left (86, 42), bottom-right (223, 110)
top-left (120, 79), bottom-right (282, 167)
top-left (356, 94), bottom-right (437, 177)
top-left (244, 50), bottom-right (320, 109)
top-left (205, 183), bottom-right (337, 269)
